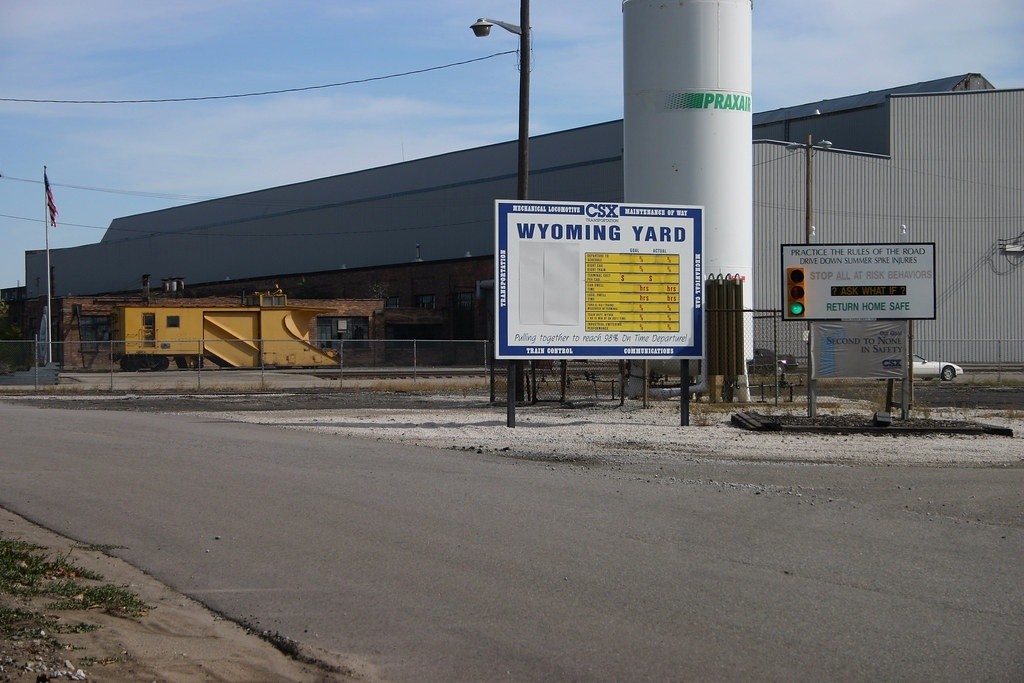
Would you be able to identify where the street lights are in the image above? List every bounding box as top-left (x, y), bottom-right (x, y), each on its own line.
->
top-left (471, 0), bottom-right (534, 427)
top-left (787, 267), bottom-right (806, 317)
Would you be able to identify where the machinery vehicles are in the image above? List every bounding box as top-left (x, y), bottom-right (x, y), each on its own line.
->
top-left (105, 283), bottom-right (344, 372)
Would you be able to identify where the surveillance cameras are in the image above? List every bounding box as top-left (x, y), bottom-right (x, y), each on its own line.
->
top-left (470, 18), bottom-right (493, 38)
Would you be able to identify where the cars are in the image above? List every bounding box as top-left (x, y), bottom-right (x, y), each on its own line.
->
top-left (907, 354), bottom-right (964, 382)
top-left (748, 349), bottom-right (799, 378)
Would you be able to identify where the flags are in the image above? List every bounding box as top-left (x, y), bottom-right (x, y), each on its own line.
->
top-left (44, 173), bottom-right (59, 228)
